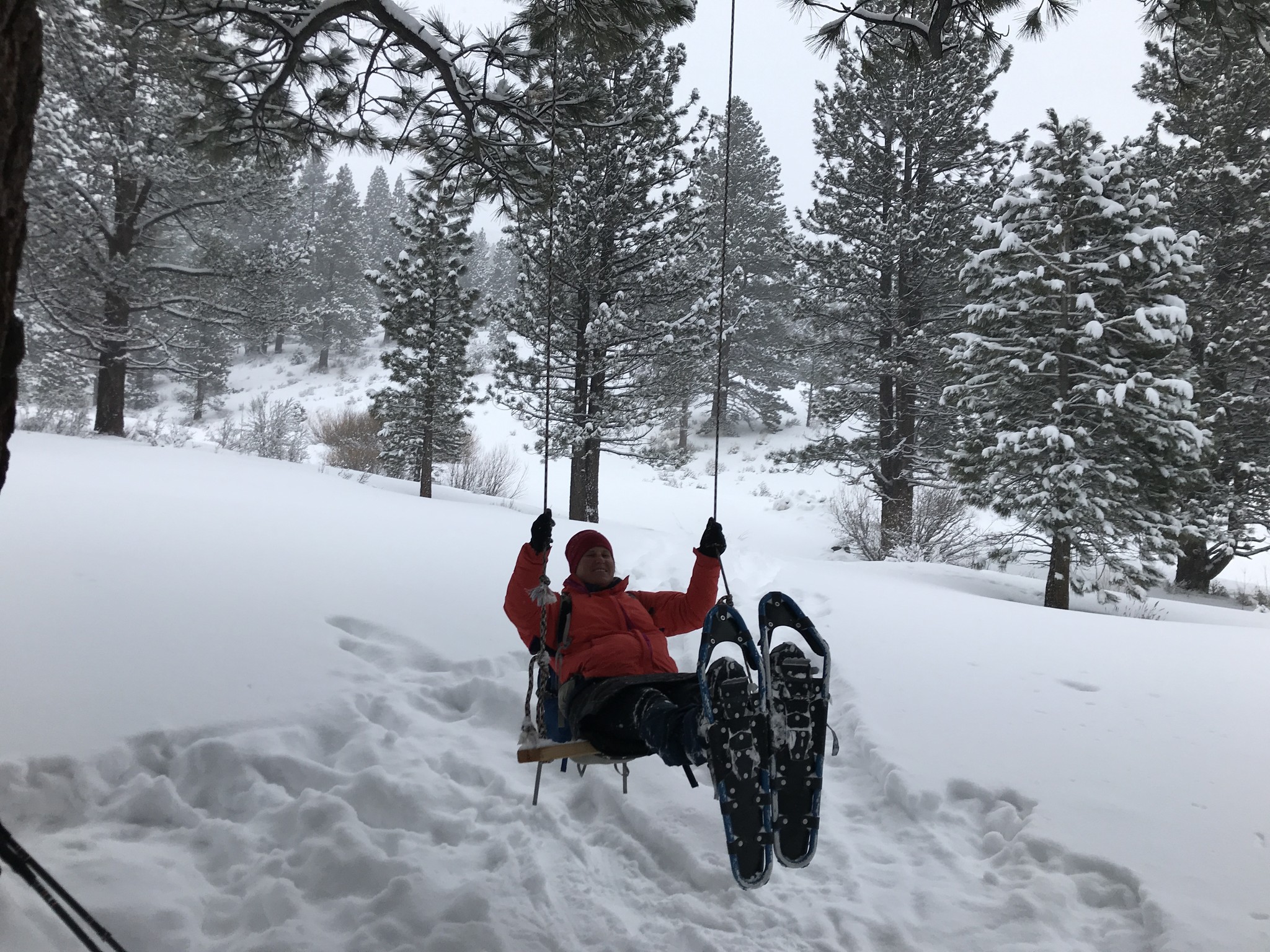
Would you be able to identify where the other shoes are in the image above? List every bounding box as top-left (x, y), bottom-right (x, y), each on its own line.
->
top-left (686, 659), bottom-right (742, 766)
top-left (766, 643), bottom-right (808, 681)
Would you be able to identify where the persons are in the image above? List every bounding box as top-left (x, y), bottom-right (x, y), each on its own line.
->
top-left (503, 508), bottom-right (839, 890)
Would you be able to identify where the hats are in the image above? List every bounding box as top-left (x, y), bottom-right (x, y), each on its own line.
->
top-left (565, 530), bottom-right (614, 574)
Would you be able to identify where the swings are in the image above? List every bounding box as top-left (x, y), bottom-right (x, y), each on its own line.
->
top-left (517, 0), bottom-right (740, 764)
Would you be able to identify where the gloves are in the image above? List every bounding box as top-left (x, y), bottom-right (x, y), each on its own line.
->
top-left (700, 517), bottom-right (726, 558)
top-left (530, 509), bottom-right (555, 552)
